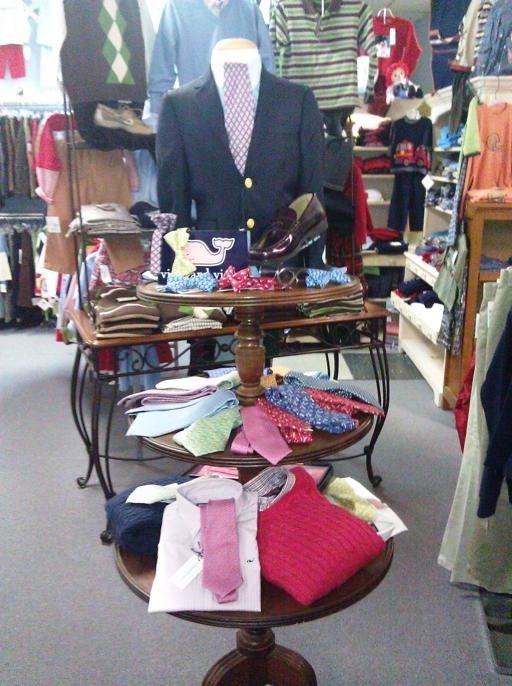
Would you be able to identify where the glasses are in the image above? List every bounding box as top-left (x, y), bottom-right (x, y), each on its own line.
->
top-left (275, 267), bottom-right (321, 292)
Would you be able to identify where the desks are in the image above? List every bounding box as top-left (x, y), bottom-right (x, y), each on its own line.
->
top-left (69, 298), bottom-right (393, 500)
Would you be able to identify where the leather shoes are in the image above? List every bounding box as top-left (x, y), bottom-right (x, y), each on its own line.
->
top-left (249, 191), bottom-right (329, 265)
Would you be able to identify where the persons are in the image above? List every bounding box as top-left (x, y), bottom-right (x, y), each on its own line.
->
top-left (374, 34), bottom-right (390, 58)
top-left (384, 61), bottom-right (422, 105)
top-left (154, 35), bottom-right (328, 289)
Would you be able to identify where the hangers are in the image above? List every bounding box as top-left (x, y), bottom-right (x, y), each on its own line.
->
top-left (1, 105), bottom-right (50, 125)
top-left (0, 214), bottom-right (46, 234)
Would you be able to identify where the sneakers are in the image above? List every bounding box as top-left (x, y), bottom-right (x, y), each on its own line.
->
top-left (93, 104), bottom-right (154, 137)
top-left (165, 269), bottom-right (215, 294)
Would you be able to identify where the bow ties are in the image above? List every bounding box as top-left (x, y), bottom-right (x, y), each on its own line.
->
top-left (149, 213), bottom-right (178, 235)
top-left (217, 266), bottom-right (250, 295)
top-left (307, 265), bottom-right (351, 289)
top-left (163, 227), bottom-right (196, 276)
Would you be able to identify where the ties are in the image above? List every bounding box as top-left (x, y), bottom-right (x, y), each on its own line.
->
top-left (116, 370), bottom-right (388, 468)
top-left (199, 498), bottom-right (242, 604)
top-left (124, 483), bottom-right (183, 506)
top-left (223, 62), bottom-right (256, 176)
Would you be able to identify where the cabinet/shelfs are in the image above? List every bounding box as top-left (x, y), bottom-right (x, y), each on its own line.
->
top-left (60, 90), bottom-right (157, 307)
top-left (423, 191), bottom-right (512, 411)
top-left (111, 276), bottom-right (395, 685)
top-left (389, 252), bottom-right (452, 407)
top-left (353, 146), bottom-right (461, 214)
